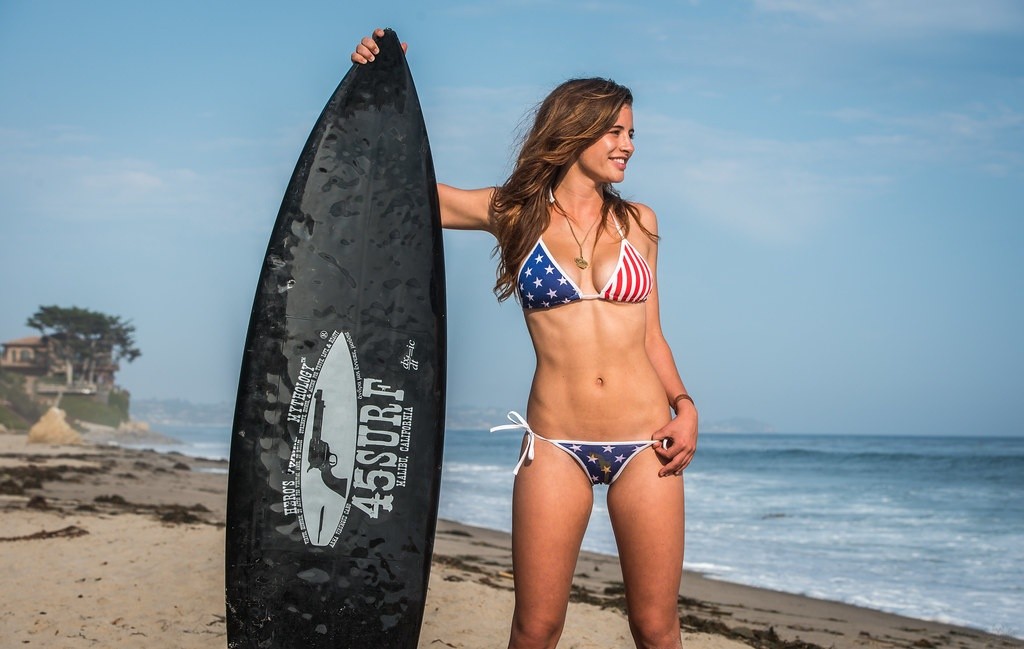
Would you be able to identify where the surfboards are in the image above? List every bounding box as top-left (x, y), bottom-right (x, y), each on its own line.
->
top-left (221, 26), bottom-right (453, 649)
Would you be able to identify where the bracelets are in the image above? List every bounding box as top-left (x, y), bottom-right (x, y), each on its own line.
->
top-left (673, 393), bottom-right (695, 415)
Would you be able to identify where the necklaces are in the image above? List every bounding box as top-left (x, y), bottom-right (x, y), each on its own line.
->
top-left (556, 201), bottom-right (600, 270)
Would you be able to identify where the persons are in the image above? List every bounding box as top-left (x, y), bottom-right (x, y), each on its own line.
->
top-left (352, 28), bottom-right (698, 649)
